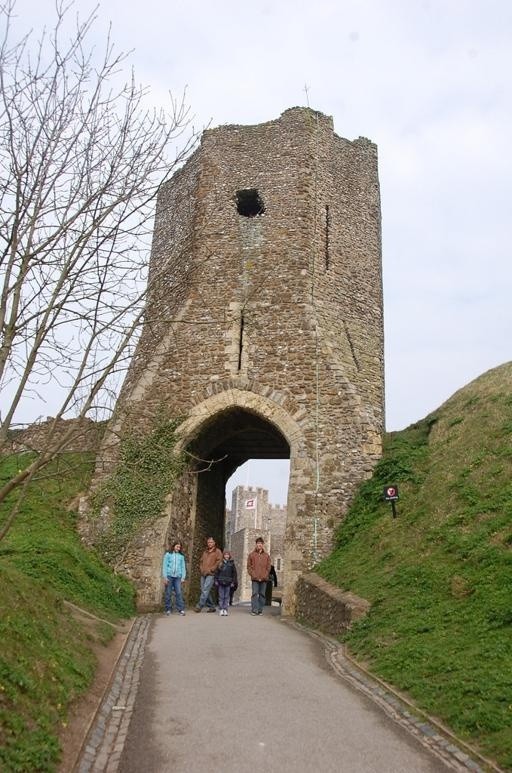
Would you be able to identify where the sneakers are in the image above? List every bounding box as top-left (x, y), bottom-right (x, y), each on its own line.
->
top-left (251, 611), bottom-right (263, 616)
top-left (219, 610), bottom-right (228, 617)
top-left (207, 606), bottom-right (216, 612)
top-left (193, 607), bottom-right (200, 612)
top-left (178, 610), bottom-right (185, 616)
top-left (166, 611), bottom-right (172, 617)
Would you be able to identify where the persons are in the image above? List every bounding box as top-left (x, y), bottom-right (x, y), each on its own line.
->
top-left (230, 559), bottom-right (239, 606)
top-left (264, 564), bottom-right (278, 606)
top-left (246, 537), bottom-right (271, 615)
top-left (161, 542), bottom-right (186, 616)
top-left (194, 535), bottom-right (223, 612)
top-left (214, 550), bottom-right (237, 615)
